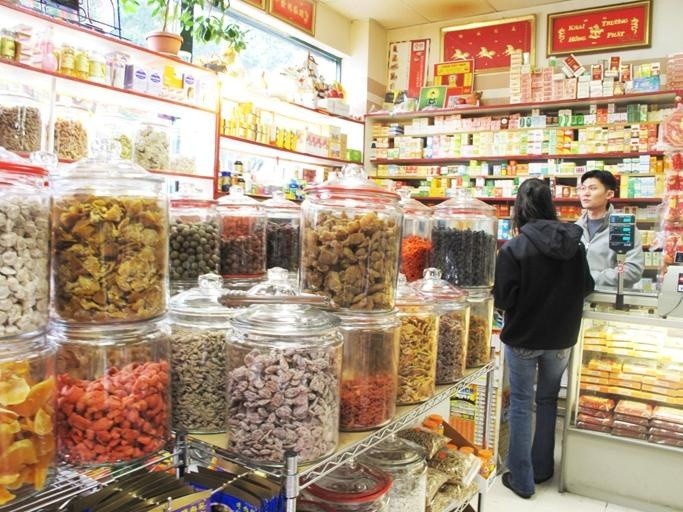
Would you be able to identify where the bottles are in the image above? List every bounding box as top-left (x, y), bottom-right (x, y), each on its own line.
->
top-left (0, 30), bottom-right (15, 59)
top-left (0, 91), bottom-right (173, 176)
top-left (52, 42), bottom-right (106, 83)
top-left (1, 162), bottom-right (498, 512)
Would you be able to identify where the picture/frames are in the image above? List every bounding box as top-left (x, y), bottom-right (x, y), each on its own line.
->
top-left (437, 1), bottom-right (653, 76)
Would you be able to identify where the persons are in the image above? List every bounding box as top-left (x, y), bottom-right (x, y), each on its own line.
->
top-left (492, 178), bottom-right (595, 499)
top-left (573, 169), bottom-right (646, 288)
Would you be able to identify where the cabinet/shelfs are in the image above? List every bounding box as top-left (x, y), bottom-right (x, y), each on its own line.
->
top-left (362, 85), bottom-right (683, 267)
top-left (187, 346), bottom-right (499, 512)
top-left (2, 2), bottom-right (365, 201)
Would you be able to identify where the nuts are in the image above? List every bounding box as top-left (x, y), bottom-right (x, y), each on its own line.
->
top-left (0, 107), bottom-right (495, 512)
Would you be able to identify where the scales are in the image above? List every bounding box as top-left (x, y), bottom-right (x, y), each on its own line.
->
top-left (584, 212), bottom-right (659, 311)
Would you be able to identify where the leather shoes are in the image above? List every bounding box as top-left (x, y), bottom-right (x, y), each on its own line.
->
top-left (533, 474), bottom-right (555, 484)
top-left (502, 472), bottom-right (533, 498)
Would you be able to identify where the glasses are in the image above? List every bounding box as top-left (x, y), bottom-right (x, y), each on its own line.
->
top-left (579, 184), bottom-right (597, 193)
